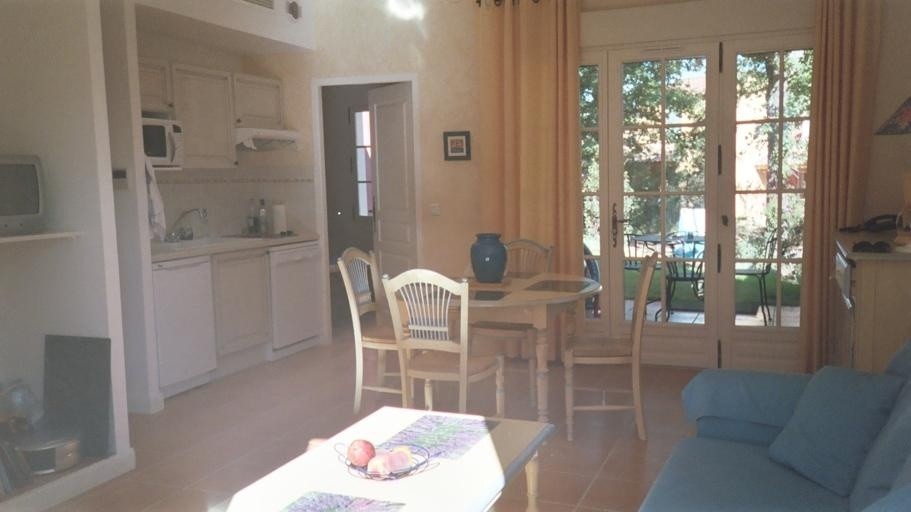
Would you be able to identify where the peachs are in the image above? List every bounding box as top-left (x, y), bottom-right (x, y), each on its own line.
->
top-left (385, 451), bottom-right (409, 475)
top-left (367, 454), bottom-right (389, 481)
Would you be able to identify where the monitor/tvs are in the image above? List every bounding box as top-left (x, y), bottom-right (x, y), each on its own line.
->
top-left (0, 153), bottom-right (44, 233)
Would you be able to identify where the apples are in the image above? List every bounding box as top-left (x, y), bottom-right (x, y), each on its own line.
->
top-left (347, 438), bottom-right (376, 467)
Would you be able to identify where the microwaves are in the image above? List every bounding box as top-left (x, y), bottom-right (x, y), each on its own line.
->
top-left (140, 117), bottom-right (184, 167)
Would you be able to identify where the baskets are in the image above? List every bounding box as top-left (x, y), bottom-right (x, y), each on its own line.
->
top-left (334, 441), bottom-right (442, 481)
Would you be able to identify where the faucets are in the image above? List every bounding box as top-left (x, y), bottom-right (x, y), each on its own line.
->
top-left (166, 208), bottom-right (207, 242)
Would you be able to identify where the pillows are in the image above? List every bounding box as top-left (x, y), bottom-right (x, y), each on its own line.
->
top-left (766, 362), bottom-right (904, 500)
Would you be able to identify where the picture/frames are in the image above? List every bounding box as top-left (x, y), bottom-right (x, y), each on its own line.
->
top-left (443, 131), bottom-right (471, 160)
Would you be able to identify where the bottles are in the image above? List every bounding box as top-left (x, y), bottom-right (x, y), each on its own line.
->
top-left (247, 198), bottom-right (268, 238)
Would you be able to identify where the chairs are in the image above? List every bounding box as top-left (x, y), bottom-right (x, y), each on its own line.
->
top-left (661, 230), bottom-right (784, 325)
top-left (638, 346), bottom-right (911, 510)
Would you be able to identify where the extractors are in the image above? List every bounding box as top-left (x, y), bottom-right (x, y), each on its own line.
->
top-left (233, 124), bottom-right (300, 153)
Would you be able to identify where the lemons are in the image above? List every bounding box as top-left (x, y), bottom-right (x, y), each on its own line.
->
top-left (395, 446), bottom-right (411, 464)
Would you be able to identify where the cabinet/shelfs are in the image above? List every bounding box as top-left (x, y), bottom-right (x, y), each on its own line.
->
top-left (0, 0), bottom-right (141, 511)
top-left (137, 61), bottom-right (176, 114)
top-left (213, 249), bottom-right (271, 356)
top-left (174, 66), bottom-right (235, 175)
top-left (271, 243), bottom-right (326, 359)
top-left (828, 228), bottom-right (911, 371)
top-left (151, 255), bottom-right (219, 398)
top-left (230, 72), bottom-right (286, 130)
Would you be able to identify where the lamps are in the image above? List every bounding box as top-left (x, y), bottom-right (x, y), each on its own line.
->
top-left (867, 95), bottom-right (910, 246)
